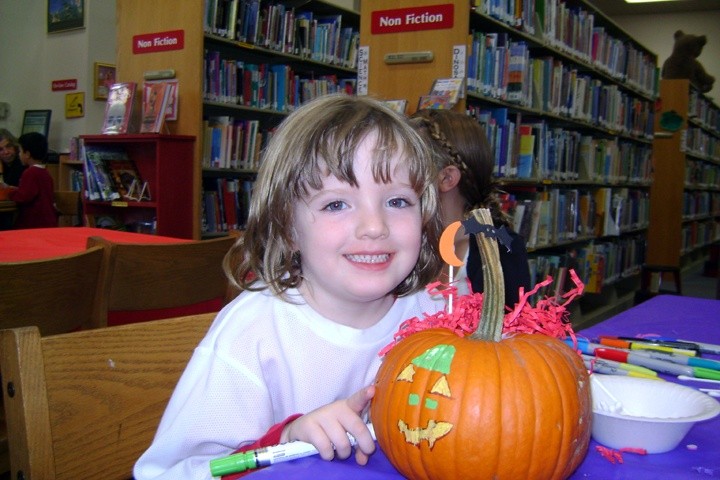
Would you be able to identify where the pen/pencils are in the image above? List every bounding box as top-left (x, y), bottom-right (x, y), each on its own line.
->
top-left (557, 334), bottom-right (720, 383)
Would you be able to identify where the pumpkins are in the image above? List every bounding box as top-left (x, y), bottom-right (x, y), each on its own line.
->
top-left (370, 207), bottom-right (592, 480)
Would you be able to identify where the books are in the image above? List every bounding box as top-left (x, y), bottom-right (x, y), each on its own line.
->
top-left (379, 0), bottom-right (720, 333)
top-left (62, 1), bottom-right (361, 241)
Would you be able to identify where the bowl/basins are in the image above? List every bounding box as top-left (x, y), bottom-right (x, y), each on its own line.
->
top-left (590, 375), bottom-right (720, 453)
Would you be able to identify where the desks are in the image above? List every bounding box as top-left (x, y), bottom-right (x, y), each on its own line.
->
top-left (0, 200), bottom-right (18, 211)
top-left (0, 224), bottom-right (223, 325)
top-left (238, 293), bottom-right (720, 480)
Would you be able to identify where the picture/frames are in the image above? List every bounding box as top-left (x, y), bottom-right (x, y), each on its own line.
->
top-left (93, 61), bottom-right (116, 101)
top-left (45, 0), bottom-right (87, 35)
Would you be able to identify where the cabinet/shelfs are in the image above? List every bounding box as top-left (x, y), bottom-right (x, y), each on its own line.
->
top-left (355, 0), bottom-right (661, 333)
top-left (116, 0), bottom-right (359, 238)
top-left (649, 76), bottom-right (720, 282)
top-left (80, 130), bottom-right (196, 238)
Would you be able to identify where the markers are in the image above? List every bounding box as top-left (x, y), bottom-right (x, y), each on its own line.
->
top-left (210, 422), bottom-right (379, 476)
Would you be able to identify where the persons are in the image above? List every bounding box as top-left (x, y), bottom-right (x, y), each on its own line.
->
top-left (0, 128), bottom-right (29, 188)
top-left (0, 131), bottom-right (60, 229)
top-left (131, 93), bottom-right (449, 480)
top-left (400, 109), bottom-right (533, 315)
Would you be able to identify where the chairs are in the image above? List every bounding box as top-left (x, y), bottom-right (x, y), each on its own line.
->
top-left (0, 236), bottom-right (111, 337)
top-left (108, 224), bottom-right (250, 319)
top-left (0, 308), bottom-right (228, 480)
top-left (53, 190), bottom-right (83, 227)
